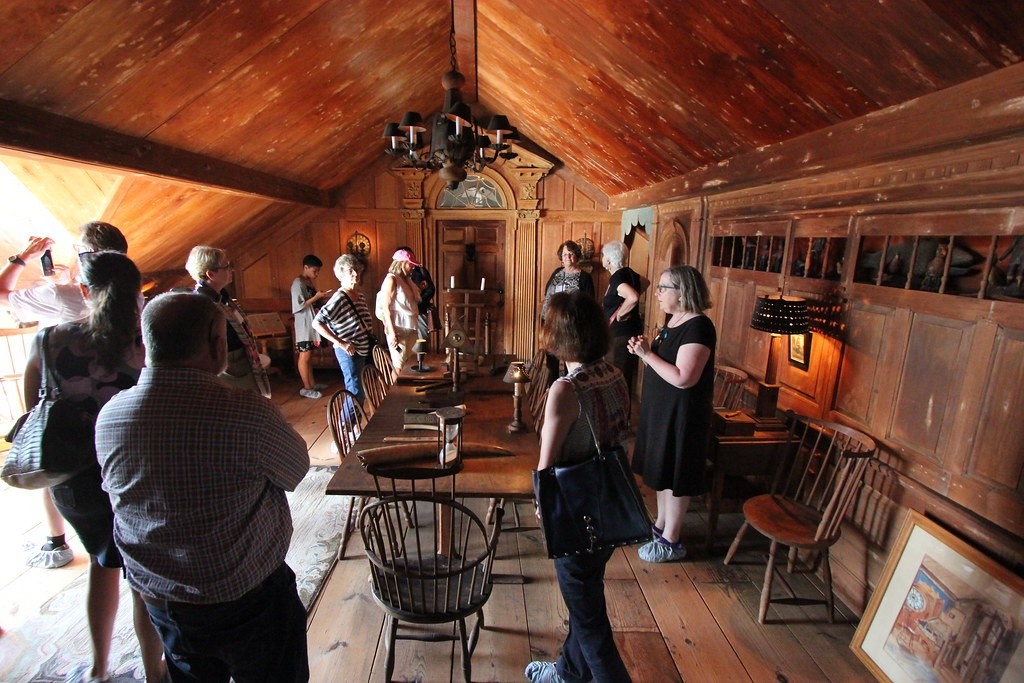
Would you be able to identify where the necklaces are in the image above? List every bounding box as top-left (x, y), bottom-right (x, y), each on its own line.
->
top-left (670, 312), bottom-right (688, 328)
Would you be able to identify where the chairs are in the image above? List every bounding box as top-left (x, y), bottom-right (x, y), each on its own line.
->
top-left (724, 417), bottom-right (877, 622)
top-left (711, 363), bottom-right (750, 409)
top-left (327, 389), bottom-right (414, 560)
top-left (360, 345), bottom-right (397, 414)
top-left (525, 350), bottom-right (559, 444)
top-left (358, 464), bottom-right (504, 683)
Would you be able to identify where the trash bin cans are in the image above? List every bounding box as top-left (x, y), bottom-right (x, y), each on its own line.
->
top-left (267, 336), bottom-right (295, 374)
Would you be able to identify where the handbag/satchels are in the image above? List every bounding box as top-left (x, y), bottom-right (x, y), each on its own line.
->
top-left (368, 333), bottom-right (380, 361)
top-left (1, 326), bottom-right (90, 490)
top-left (531, 376), bottom-right (652, 560)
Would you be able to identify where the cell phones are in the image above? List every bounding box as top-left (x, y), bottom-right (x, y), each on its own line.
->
top-left (327, 289), bottom-right (332, 293)
top-left (41, 249), bottom-right (56, 276)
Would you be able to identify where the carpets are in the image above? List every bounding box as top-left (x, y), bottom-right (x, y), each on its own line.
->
top-left (0, 464), bottom-right (370, 683)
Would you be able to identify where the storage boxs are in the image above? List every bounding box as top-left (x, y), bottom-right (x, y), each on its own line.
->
top-left (714, 412), bottom-right (754, 434)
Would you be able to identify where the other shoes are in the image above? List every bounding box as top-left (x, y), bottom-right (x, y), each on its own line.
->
top-left (299, 388), bottom-right (322, 399)
top-left (638, 540), bottom-right (687, 562)
top-left (331, 422), bottom-right (363, 454)
top-left (20, 542), bottom-right (74, 569)
top-left (311, 384), bottom-right (329, 389)
top-left (525, 660), bottom-right (566, 683)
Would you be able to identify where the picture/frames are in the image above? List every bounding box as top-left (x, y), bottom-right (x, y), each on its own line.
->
top-left (788, 334), bottom-right (810, 369)
top-left (848, 508), bottom-right (1024, 683)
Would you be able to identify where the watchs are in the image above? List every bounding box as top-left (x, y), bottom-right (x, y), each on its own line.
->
top-left (8, 255), bottom-right (26, 266)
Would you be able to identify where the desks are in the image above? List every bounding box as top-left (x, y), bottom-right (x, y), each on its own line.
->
top-left (707, 410), bottom-right (800, 551)
top-left (325, 353), bottom-right (543, 498)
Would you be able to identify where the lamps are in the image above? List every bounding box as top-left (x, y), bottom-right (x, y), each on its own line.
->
top-left (410, 340), bottom-right (431, 372)
top-left (748, 296), bottom-right (811, 429)
top-left (503, 362), bottom-right (531, 432)
top-left (383, 0), bottom-right (521, 192)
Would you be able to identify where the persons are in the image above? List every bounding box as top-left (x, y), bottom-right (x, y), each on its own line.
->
top-left (384, 250), bottom-right (418, 385)
top-left (290, 255), bottom-right (329, 398)
top-left (23, 221), bottom-right (129, 571)
top-left (183, 243), bottom-right (272, 395)
top-left (601, 241), bottom-right (651, 444)
top-left (94, 292), bottom-right (310, 683)
top-left (0, 251), bottom-right (170, 683)
top-left (525, 290), bottom-right (634, 683)
top-left (627, 265), bottom-right (716, 562)
top-left (312, 254), bottom-right (377, 455)
top-left (540, 241), bottom-right (594, 389)
top-left (392, 246), bottom-right (436, 353)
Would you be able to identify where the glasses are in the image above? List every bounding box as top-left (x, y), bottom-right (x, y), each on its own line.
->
top-left (212, 260), bottom-right (232, 269)
top-left (656, 284), bottom-right (676, 291)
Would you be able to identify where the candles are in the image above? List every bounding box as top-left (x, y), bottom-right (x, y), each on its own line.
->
top-left (480, 278), bottom-right (486, 291)
top-left (450, 275), bottom-right (455, 288)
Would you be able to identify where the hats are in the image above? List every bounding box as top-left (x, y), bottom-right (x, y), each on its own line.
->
top-left (392, 248), bottom-right (420, 265)
